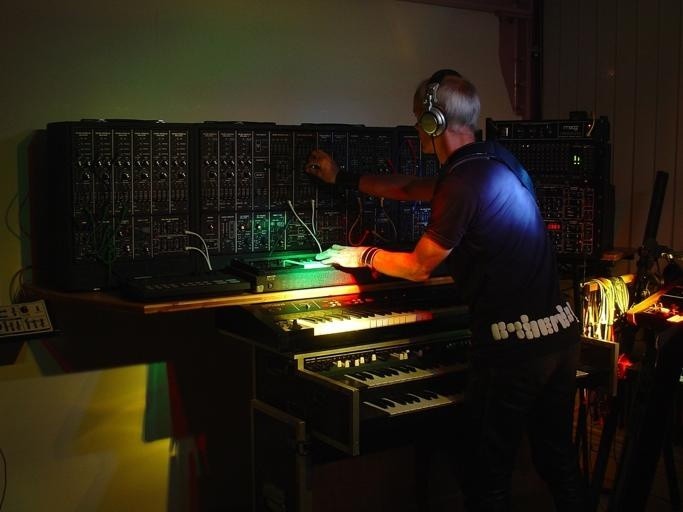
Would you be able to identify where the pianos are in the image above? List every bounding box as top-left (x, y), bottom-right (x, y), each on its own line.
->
top-left (252, 281), bottom-right (604, 457)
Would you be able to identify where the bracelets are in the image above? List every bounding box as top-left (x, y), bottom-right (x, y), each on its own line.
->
top-left (336, 170), bottom-right (361, 191)
top-left (361, 247), bottom-right (376, 266)
top-left (366, 249), bottom-right (380, 269)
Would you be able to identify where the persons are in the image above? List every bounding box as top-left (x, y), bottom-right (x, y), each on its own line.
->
top-left (304, 69), bottom-right (582, 512)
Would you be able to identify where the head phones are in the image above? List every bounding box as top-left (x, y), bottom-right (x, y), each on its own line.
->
top-left (419, 69), bottom-right (462, 138)
